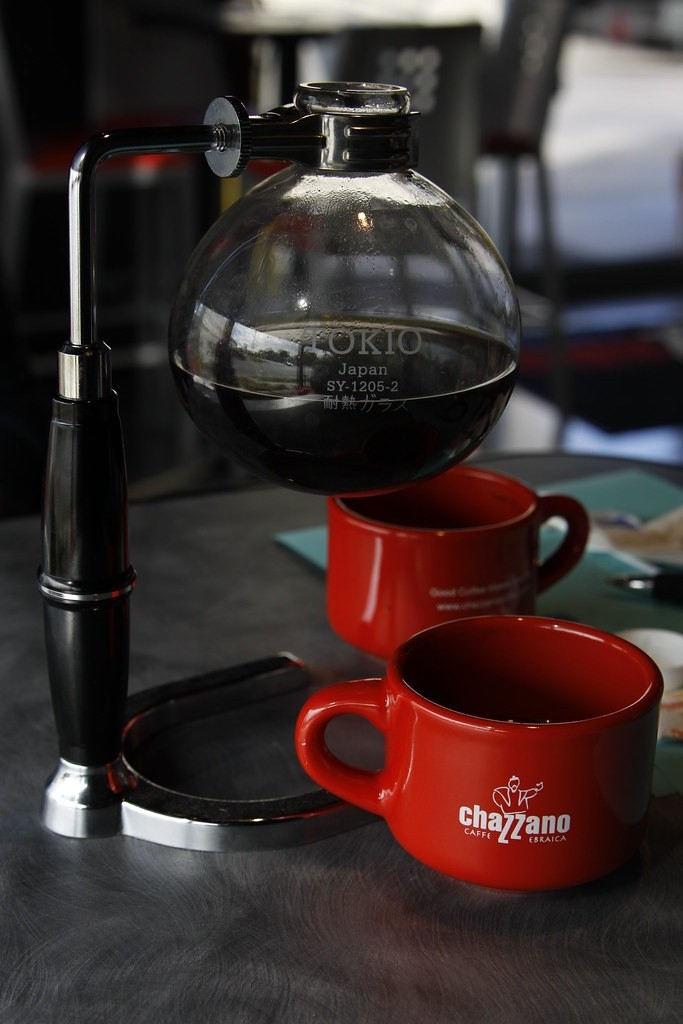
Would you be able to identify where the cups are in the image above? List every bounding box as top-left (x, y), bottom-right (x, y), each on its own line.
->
top-left (291, 612), bottom-right (665, 893)
top-left (326, 466), bottom-right (589, 664)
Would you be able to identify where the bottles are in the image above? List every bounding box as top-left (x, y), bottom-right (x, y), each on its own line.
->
top-left (168, 81), bottom-right (523, 497)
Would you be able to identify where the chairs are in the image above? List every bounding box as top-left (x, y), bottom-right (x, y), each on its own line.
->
top-left (328, 0), bottom-right (617, 335)
top-left (1, 1), bottom-right (204, 370)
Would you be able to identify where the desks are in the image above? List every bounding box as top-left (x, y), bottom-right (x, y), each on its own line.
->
top-left (205, 17), bottom-right (492, 264)
top-left (0, 452), bottom-right (683, 1024)
